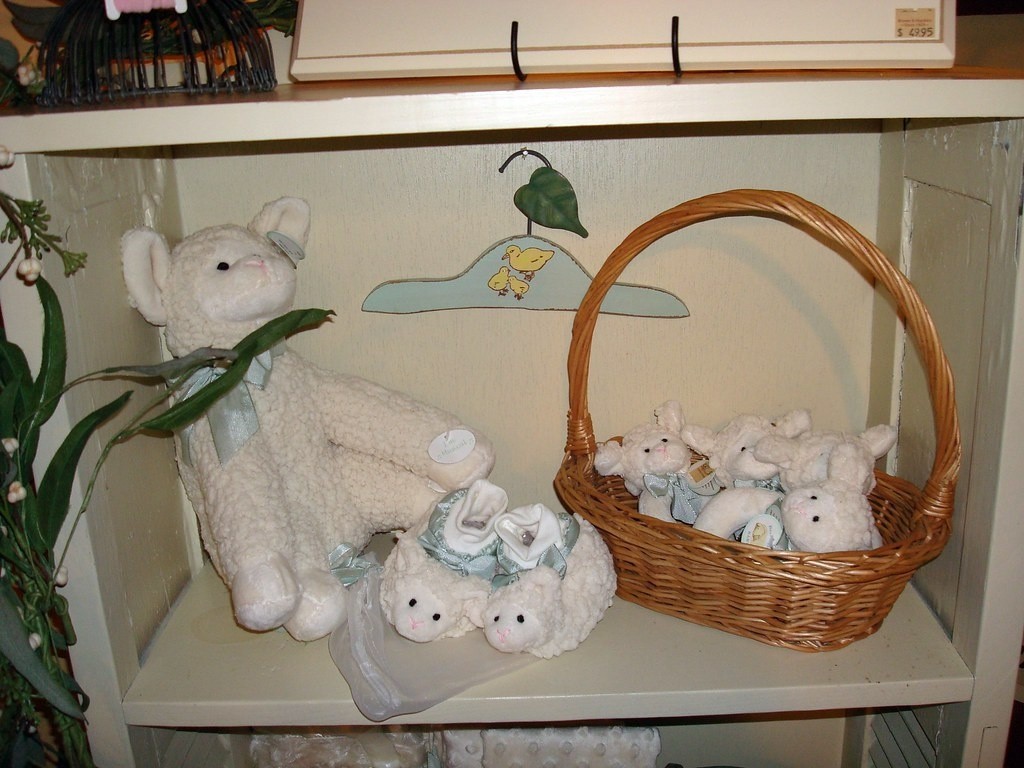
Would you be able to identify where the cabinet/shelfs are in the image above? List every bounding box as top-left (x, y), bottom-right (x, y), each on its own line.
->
top-left (0, 63), bottom-right (1024, 768)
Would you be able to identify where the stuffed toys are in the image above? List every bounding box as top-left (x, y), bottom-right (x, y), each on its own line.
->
top-left (594, 400), bottom-right (898, 552)
top-left (121, 197), bottom-right (619, 663)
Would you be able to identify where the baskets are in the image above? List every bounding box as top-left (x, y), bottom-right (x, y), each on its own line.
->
top-left (554, 188), bottom-right (961, 652)
